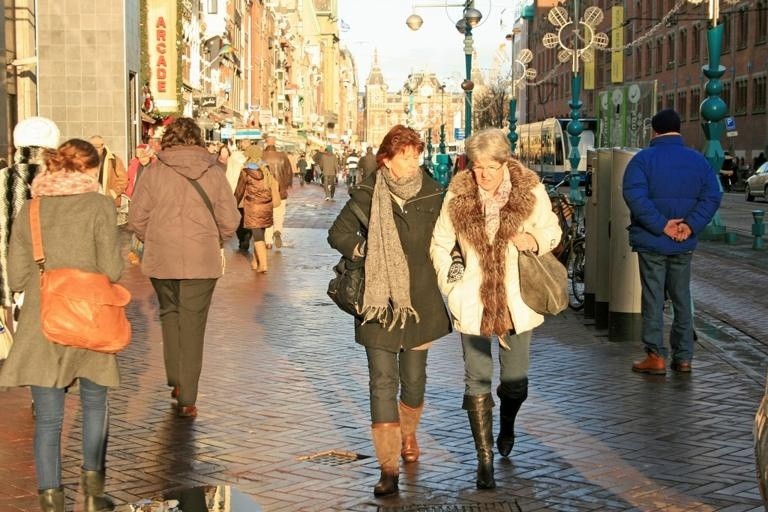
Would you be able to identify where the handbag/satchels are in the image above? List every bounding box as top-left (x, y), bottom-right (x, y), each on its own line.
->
top-left (29, 195), bottom-right (131, 353)
top-left (188, 178), bottom-right (226, 275)
top-left (328, 200), bottom-right (393, 322)
top-left (519, 249), bottom-right (569, 315)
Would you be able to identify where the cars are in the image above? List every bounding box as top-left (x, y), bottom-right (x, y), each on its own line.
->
top-left (745, 161), bottom-right (768, 201)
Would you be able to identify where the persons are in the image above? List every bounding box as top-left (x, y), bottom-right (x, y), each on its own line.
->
top-left (735, 156), bottom-right (740, 179)
top-left (262, 144), bottom-right (293, 250)
top-left (429, 127), bottom-right (562, 491)
top-left (214, 140), bottom-right (231, 166)
top-left (0, 116), bottom-right (62, 336)
top-left (737, 157), bottom-right (747, 177)
top-left (719, 152), bottom-right (735, 192)
top-left (358, 147), bottom-right (377, 182)
top-left (311, 148), bottom-right (321, 184)
top-left (0, 139), bottom-right (125, 512)
top-left (754, 152), bottom-right (768, 170)
top-left (325, 126), bottom-right (466, 498)
top-left (296, 154), bottom-right (308, 187)
top-left (320, 146), bottom-right (338, 201)
top-left (622, 110), bottom-right (724, 374)
top-left (119, 143), bottom-right (158, 267)
top-left (346, 149), bottom-right (359, 186)
top-left (225, 140), bottom-right (251, 254)
top-left (128, 117), bottom-right (242, 418)
top-left (305, 152), bottom-right (315, 184)
top-left (90, 137), bottom-right (128, 208)
top-left (233, 145), bottom-right (274, 273)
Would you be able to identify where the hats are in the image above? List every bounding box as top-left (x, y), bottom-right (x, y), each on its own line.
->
top-left (136, 145), bottom-right (153, 157)
top-left (13, 118), bottom-right (60, 149)
top-left (652, 109), bottom-right (681, 133)
top-left (244, 146), bottom-right (262, 158)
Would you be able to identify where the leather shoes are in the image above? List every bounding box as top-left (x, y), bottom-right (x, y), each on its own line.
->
top-left (242, 233), bottom-right (251, 249)
top-left (239, 242), bottom-right (243, 249)
top-left (266, 244), bottom-right (271, 249)
top-left (178, 406), bottom-right (198, 417)
top-left (633, 353), bottom-right (666, 375)
top-left (671, 358), bottom-right (691, 372)
top-left (274, 231), bottom-right (281, 248)
top-left (171, 386), bottom-right (180, 398)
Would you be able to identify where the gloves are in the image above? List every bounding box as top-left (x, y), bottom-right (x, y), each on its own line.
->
top-left (448, 257), bottom-right (464, 282)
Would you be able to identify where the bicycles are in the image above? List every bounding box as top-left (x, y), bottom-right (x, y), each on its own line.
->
top-left (536, 172), bottom-right (592, 312)
top-left (727, 173), bottom-right (732, 192)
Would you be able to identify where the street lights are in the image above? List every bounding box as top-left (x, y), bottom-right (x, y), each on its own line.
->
top-left (504, 17), bottom-right (522, 159)
top-left (407, 0), bottom-right (483, 148)
top-left (433, 84), bottom-right (454, 188)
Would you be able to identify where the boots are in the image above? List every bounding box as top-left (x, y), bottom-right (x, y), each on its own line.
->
top-left (497, 398), bottom-right (525, 456)
top-left (399, 399), bottom-right (423, 462)
top-left (462, 393), bottom-right (495, 488)
top-left (81, 467), bottom-right (114, 512)
top-left (371, 423), bottom-right (401, 495)
top-left (255, 241), bottom-right (267, 272)
top-left (250, 248), bottom-right (259, 269)
top-left (39, 486), bottom-right (65, 512)
